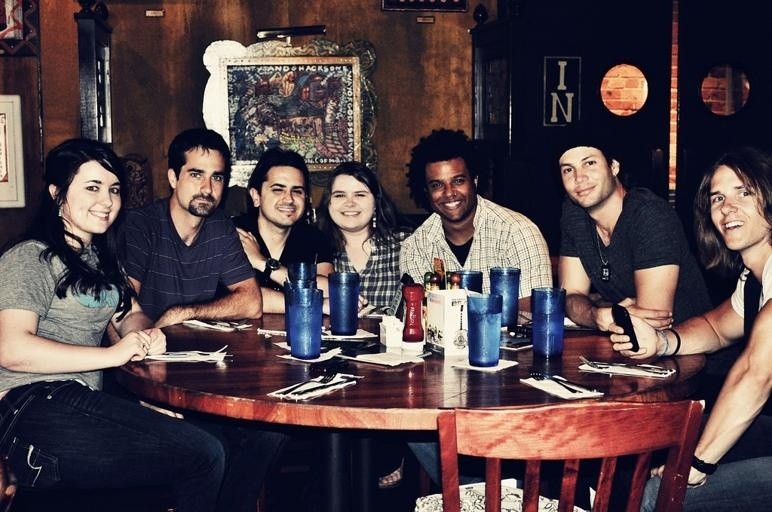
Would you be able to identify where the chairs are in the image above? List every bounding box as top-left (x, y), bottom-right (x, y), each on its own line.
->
top-left (414, 402), bottom-right (705, 512)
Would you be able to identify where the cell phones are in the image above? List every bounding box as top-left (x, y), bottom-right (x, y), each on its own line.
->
top-left (612, 303), bottom-right (639, 352)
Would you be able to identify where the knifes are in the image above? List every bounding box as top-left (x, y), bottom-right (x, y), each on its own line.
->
top-left (293, 377), bottom-right (355, 396)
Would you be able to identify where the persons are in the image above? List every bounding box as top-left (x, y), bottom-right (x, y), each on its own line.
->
top-left (316, 162), bottom-right (414, 490)
top-left (609, 147), bottom-right (772, 512)
top-left (216, 147), bottom-right (368, 315)
top-left (538, 118), bottom-right (715, 511)
top-left (398, 127), bottom-right (553, 489)
top-left (105, 129), bottom-right (289, 512)
top-left (0, 138), bottom-right (225, 512)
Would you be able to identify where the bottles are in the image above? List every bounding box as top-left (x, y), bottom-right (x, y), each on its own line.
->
top-left (404, 272), bottom-right (459, 342)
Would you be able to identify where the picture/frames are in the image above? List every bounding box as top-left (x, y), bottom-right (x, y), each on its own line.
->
top-left (0, 94), bottom-right (26, 209)
top-left (381, 0), bottom-right (468, 13)
top-left (201, 37), bottom-right (379, 191)
top-left (0, 0), bottom-right (40, 57)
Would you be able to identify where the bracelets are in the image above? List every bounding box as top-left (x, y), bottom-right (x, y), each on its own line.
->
top-left (655, 329), bottom-right (669, 356)
top-left (664, 328), bottom-right (680, 357)
top-left (687, 477), bottom-right (706, 486)
top-left (691, 455), bottom-right (719, 475)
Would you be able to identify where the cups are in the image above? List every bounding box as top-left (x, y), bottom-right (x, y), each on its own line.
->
top-left (491, 266), bottom-right (521, 328)
top-left (458, 270), bottom-right (483, 293)
top-left (531, 287), bottom-right (566, 358)
top-left (284, 261), bottom-right (360, 358)
top-left (467, 293), bottom-right (500, 366)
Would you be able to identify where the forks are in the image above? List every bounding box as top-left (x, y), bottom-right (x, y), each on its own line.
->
top-left (275, 369), bottom-right (338, 395)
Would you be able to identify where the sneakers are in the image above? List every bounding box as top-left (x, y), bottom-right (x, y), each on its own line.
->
top-left (378, 455), bottom-right (407, 488)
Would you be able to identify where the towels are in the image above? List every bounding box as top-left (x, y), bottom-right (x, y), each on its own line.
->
top-left (268, 372), bottom-right (362, 400)
top-left (184, 320), bottom-right (251, 332)
top-left (521, 375), bottom-right (605, 399)
top-left (145, 350), bottom-right (225, 363)
top-left (580, 364), bottom-right (676, 378)
